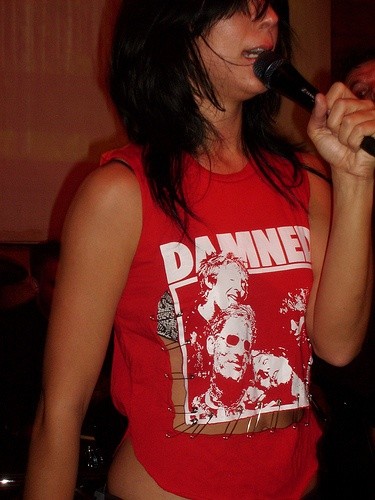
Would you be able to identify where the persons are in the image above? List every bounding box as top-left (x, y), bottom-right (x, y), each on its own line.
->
top-left (310, 49), bottom-right (375, 500)
top-left (25, 0), bottom-right (375, 500)
top-left (0, 239), bottom-right (127, 500)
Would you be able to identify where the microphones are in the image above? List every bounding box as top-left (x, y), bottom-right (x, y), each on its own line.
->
top-left (253, 49), bottom-right (375, 157)
